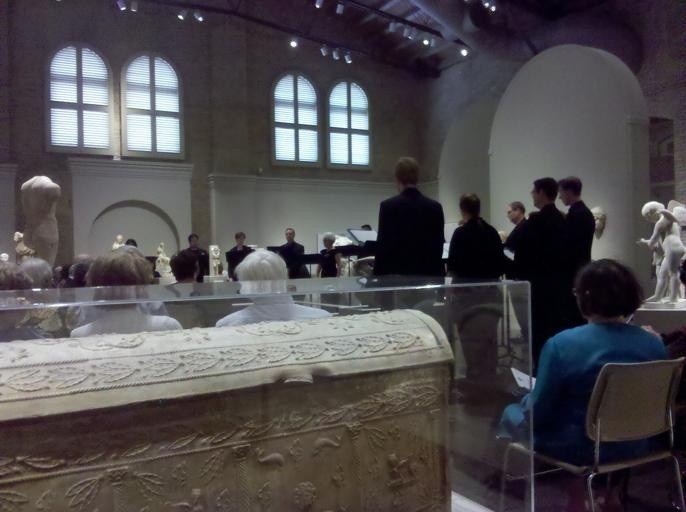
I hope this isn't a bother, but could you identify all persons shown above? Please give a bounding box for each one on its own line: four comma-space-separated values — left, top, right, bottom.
58, 264, 85, 302
446, 194, 503, 416
70, 252, 183, 338
20, 258, 67, 338
77, 246, 167, 326
503, 200, 529, 345
483, 261, 667, 499
590, 207, 606, 231
226, 232, 255, 282
113, 234, 126, 251
165, 251, 199, 296
516, 176, 565, 378
0, 263, 45, 342
357, 225, 376, 259
365, 157, 444, 313
316, 232, 342, 314
278, 227, 305, 280
51, 267, 63, 287
183, 234, 210, 283
635, 200, 686, 303
215, 250, 331, 328
558, 177, 596, 329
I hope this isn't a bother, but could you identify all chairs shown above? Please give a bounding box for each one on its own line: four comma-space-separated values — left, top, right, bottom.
497, 353, 686, 512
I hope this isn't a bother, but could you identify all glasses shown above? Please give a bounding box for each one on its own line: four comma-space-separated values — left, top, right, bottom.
572, 287, 580, 297
